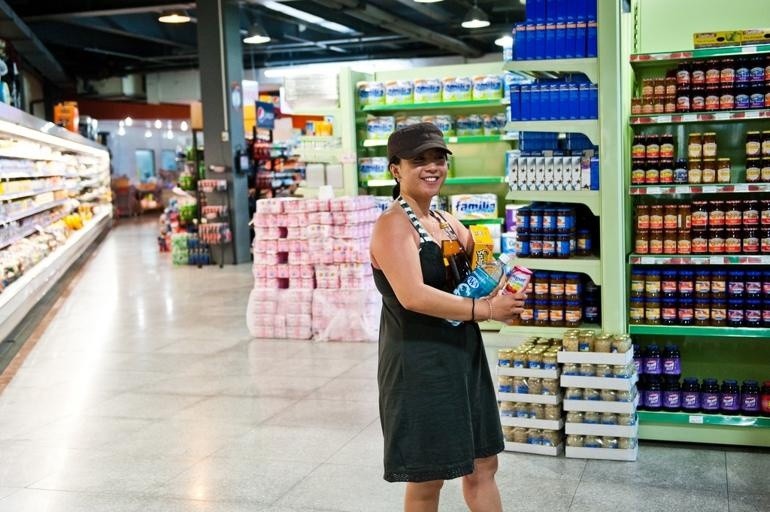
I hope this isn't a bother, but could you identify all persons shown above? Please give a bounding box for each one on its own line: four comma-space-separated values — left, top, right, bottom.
370, 123, 527, 512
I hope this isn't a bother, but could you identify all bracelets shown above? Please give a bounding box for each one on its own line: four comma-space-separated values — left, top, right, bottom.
485, 298, 493, 320
472, 297, 475, 321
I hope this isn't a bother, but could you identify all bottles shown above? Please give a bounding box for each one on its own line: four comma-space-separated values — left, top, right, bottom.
445, 253, 511, 326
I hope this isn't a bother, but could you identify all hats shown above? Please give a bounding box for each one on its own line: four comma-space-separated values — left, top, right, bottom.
387, 122, 452, 163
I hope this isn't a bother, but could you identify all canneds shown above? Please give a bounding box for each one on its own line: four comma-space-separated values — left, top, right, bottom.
629, 55, 770, 327
505, 202, 598, 328
497, 330, 770, 449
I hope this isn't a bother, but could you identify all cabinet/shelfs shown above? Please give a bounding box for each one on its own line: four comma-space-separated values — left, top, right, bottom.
0, 103, 115, 344
493, 0, 769, 465
278, 61, 508, 331
175, 83, 307, 270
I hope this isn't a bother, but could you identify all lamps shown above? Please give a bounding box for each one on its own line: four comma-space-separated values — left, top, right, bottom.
155, 11, 194, 28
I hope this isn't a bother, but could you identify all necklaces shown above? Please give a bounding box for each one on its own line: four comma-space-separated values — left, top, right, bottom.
430, 215, 435, 236
